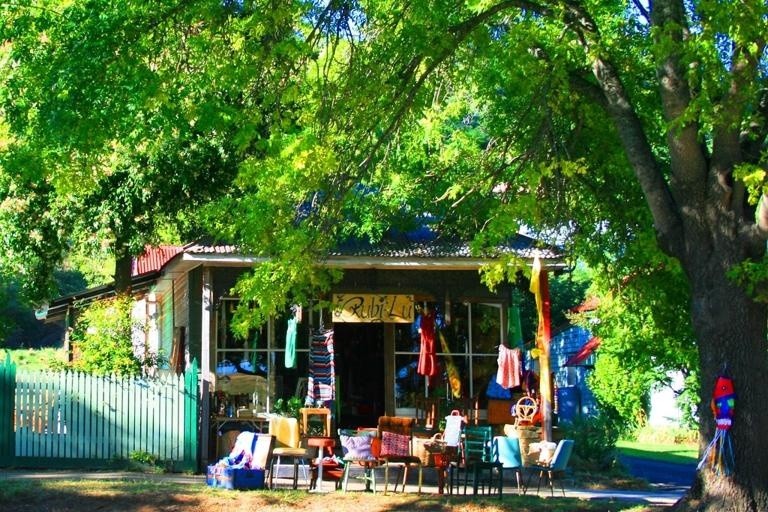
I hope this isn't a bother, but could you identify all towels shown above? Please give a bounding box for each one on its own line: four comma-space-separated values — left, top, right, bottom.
494, 340, 524, 393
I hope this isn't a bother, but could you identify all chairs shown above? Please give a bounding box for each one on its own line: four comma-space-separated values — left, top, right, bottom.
262, 393, 581, 502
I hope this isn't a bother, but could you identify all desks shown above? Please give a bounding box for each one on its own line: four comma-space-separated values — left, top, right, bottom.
208, 413, 270, 464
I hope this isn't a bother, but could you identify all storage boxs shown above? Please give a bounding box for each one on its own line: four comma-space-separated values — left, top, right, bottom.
203, 428, 278, 495
